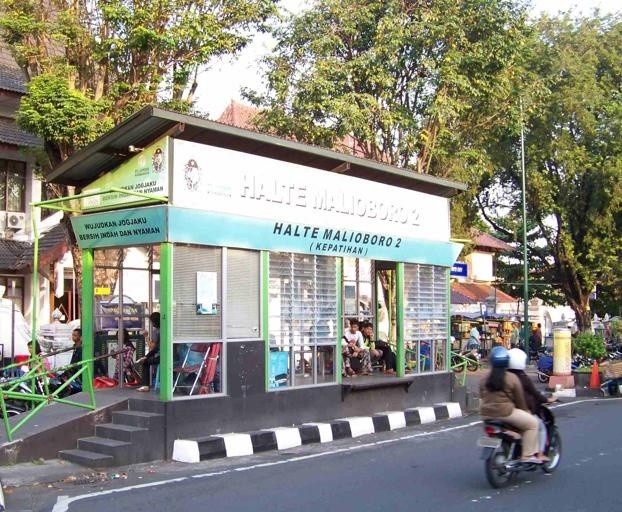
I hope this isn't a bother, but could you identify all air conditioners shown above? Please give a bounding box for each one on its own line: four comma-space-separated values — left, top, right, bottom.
6, 212, 24, 230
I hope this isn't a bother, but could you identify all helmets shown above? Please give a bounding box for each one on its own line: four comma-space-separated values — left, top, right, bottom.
489, 346, 509, 367
505, 348, 528, 370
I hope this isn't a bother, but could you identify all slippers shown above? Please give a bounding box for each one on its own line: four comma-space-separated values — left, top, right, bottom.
520, 455, 550, 464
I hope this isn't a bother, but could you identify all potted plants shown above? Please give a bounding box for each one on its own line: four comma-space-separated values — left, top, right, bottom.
572, 366, 604, 387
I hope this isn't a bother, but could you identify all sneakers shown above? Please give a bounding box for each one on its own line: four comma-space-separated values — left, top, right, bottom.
136, 386, 150, 391
342, 365, 410, 376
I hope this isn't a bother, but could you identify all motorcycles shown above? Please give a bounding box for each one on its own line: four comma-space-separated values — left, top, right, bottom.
451, 335, 622, 384
476, 384, 562, 489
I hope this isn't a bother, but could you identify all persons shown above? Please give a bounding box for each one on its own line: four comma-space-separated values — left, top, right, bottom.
68, 328, 82, 393
136, 312, 160, 392
528, 331, 541, 350
469, 323, 481, 352
479, 346, 543, 467
342, 320, 384, 377
25, 340, 55, 377
509, 321, 521, 348
508, 344, 559, 463
110, 328, 137, 383
535, 323, 543, 346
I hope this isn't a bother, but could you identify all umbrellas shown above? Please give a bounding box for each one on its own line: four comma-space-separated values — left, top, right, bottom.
197, 343, 221, 394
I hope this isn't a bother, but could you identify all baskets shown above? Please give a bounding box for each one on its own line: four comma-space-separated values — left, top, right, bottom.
599, 359, 622, 379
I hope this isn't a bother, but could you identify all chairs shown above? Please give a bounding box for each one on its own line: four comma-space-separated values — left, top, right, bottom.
149, 343, 210, 395
